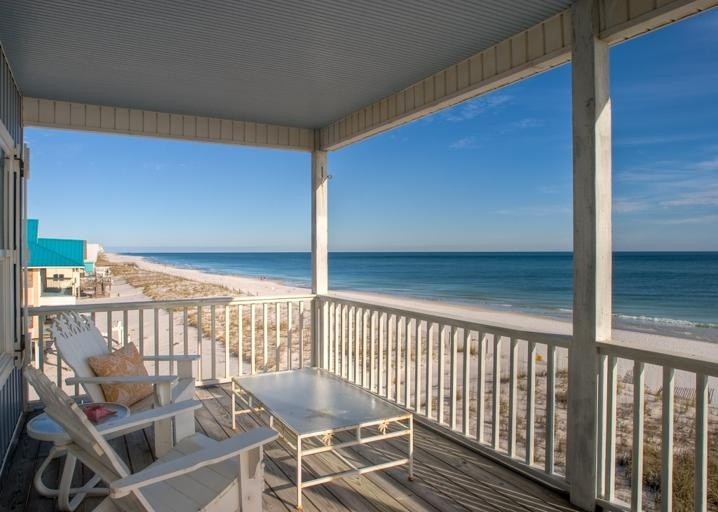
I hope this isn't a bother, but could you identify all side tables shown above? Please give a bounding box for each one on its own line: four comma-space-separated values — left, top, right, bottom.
19, 401, 132, 512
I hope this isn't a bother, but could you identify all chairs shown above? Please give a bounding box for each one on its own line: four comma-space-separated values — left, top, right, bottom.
18, 363, 280, 512
46, 308, 201, 446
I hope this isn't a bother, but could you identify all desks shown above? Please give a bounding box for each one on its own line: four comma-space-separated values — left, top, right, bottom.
231, 365, 416, 510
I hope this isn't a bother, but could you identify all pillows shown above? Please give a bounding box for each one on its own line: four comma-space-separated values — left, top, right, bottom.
86, 341, 154, 407
